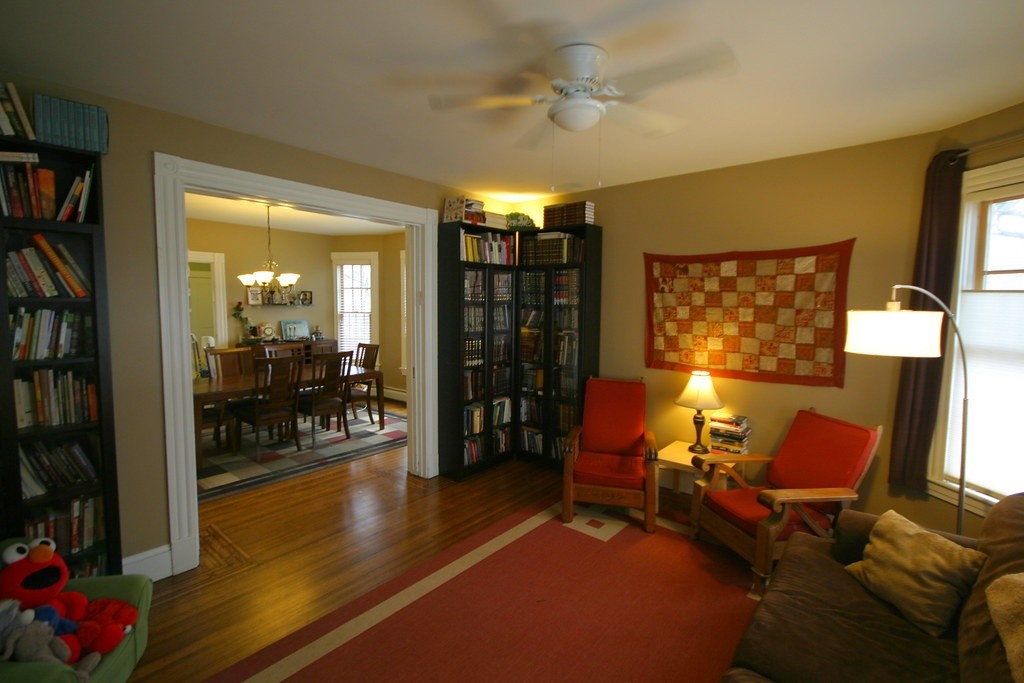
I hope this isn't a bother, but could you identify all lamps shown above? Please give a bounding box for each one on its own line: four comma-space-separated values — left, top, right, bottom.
674, 371, 724, 455
531, 44, 625, 132
236, 204, 301, 303
843, 284, 969, 534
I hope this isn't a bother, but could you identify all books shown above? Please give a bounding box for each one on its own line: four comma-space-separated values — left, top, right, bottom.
444, 195, 515, 467
709, 413, 752, 456
518, 232, 588, 462
0, 81, 109, 583
543, 200, 595, 227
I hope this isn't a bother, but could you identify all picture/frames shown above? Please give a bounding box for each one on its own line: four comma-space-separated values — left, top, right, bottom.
246, 287, 263, 306
300, 291, 313, 306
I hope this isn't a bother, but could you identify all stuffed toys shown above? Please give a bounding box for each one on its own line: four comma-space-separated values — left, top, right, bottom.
0, 540, 139, 683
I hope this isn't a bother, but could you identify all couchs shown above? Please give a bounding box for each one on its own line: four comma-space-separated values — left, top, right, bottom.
0, 567, 152, 683
720, 490, 1024, 683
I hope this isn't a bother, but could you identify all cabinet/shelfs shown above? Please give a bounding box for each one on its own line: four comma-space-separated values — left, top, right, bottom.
0, 134, 123, 585
437, 222, 602, 481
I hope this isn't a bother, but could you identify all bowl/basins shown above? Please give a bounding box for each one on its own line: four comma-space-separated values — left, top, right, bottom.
242, 337, 262, 345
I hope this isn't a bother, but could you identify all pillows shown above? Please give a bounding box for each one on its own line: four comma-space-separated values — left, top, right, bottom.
844, 509, 989, 638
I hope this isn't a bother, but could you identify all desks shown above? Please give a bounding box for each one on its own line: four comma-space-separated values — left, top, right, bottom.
193, 364, 385, 470
206, 339, 338, 376
648, 441, 745, 540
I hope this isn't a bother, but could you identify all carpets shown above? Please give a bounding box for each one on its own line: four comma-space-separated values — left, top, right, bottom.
195, 402, 407, 504
203, 491, 775, 683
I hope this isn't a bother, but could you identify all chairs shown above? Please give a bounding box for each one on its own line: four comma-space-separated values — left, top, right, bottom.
207, 343, 380, 456
561, 377, 662, 532
687, 409, 883, 596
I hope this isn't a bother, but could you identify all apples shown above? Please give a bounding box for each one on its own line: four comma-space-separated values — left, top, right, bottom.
247, 335, 255, 340
